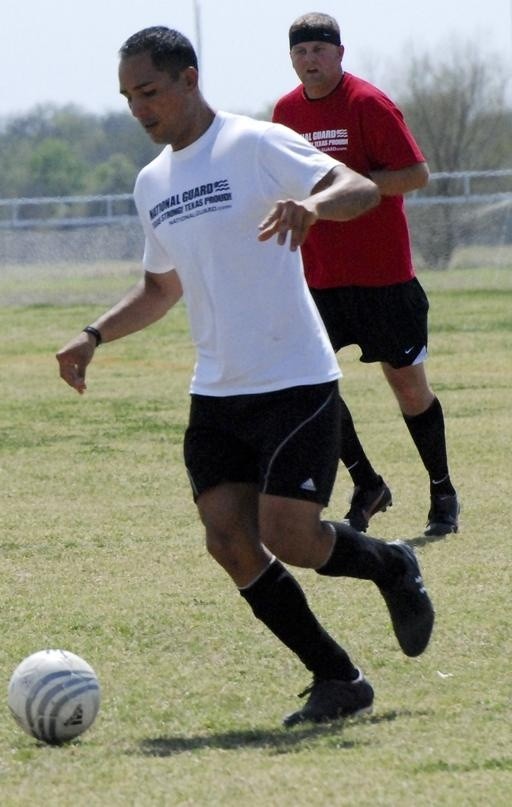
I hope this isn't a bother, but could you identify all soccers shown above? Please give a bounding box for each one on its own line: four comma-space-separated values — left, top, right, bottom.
8, 650, 98, 744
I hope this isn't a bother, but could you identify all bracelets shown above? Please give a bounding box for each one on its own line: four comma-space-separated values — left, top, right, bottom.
80, 324, 106, 349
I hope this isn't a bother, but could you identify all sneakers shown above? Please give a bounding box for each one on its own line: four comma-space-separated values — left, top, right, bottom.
284, 666, 374, 728
379, 539, 432, 656
344, 476, 391, 531
424, 493, 457, 538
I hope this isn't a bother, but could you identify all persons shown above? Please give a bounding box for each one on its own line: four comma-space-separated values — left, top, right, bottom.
54, 24, 435, 730
269, 11, 463, 540
